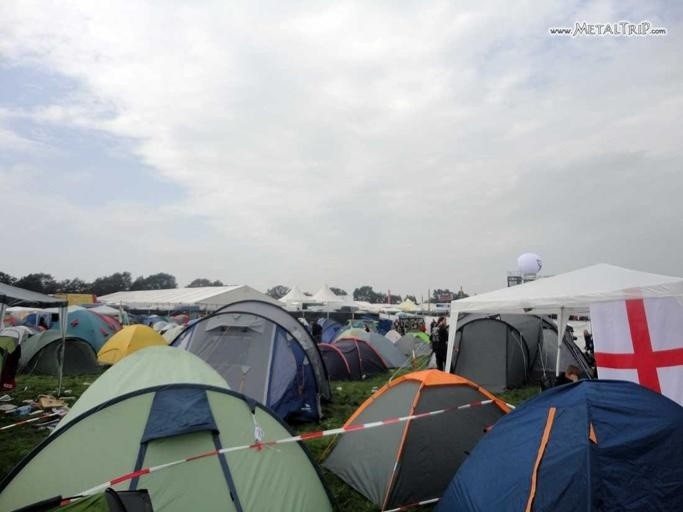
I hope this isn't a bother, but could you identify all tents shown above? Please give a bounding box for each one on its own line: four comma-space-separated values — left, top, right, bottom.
0, 298, 683, 512
